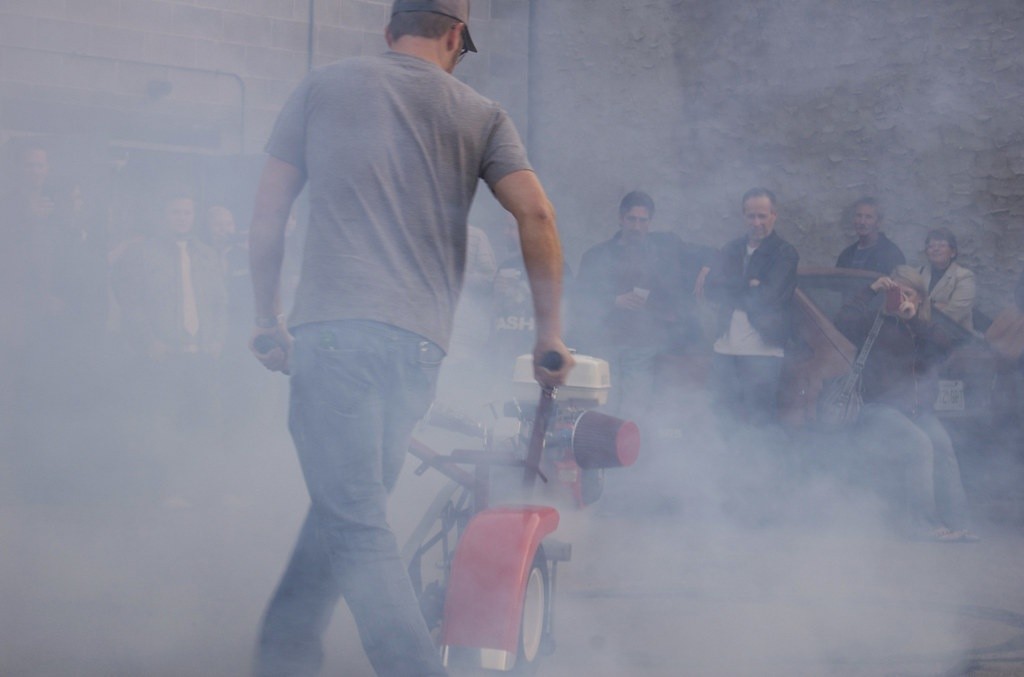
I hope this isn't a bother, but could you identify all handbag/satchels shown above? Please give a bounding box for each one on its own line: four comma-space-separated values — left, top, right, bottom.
819, 372, 864, 431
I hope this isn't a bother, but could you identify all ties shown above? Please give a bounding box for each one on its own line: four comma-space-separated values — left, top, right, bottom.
176, 239, 200, 336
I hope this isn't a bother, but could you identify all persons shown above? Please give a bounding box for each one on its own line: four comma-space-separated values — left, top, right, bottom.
244, 0, 575, 677
578, 188, 716, 420
913, 226, 977, 335
703, 186, 800, 432
487, 198, 604, 381
834, 264, 981, 544
835, 194, 907, 279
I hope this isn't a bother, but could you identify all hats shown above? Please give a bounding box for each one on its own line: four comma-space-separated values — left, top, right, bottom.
392, 0, 478, 54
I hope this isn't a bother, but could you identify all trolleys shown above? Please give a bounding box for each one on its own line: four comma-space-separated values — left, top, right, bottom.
252, 332, 640, 677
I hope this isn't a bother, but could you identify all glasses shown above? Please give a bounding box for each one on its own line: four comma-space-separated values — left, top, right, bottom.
927, 242, 950, 248
452, 19, 469, 65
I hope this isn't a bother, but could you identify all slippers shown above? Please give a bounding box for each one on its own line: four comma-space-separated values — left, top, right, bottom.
957, 534, 982, 541
913, 528, 963, 543
